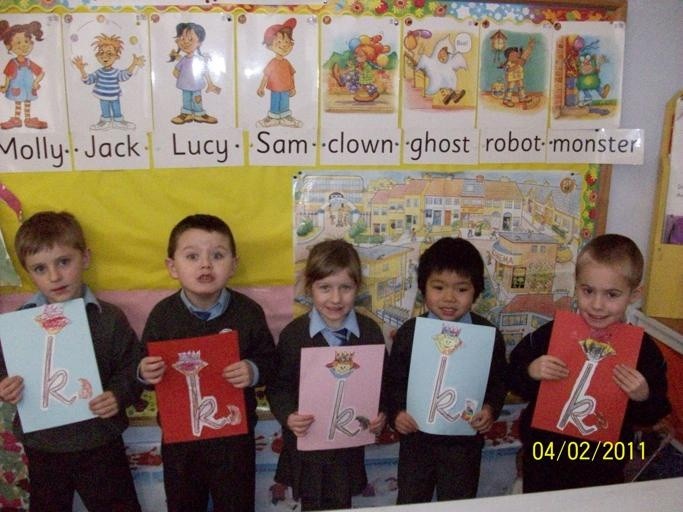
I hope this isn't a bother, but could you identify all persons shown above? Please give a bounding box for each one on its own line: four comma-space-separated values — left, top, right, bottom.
134, 214, 279, 512
386, 237, 509, 507
264, 239, 390, 511
508, 233, 671, 495
0, 210, 142, 510
